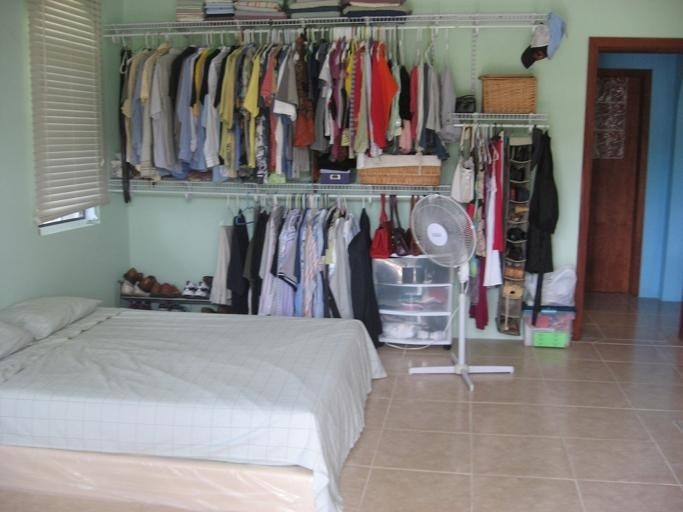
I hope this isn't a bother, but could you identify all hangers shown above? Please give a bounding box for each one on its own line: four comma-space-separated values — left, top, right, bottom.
223, 192, 365, 228
456, 121, 502, 168
111, 23, 449, 74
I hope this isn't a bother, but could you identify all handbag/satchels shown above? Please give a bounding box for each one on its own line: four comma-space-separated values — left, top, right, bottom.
455, 94, 476, 113
401, 267, 424, 284
387, 194, 410, 255
368, 194, 393, 259
449, 126, 476, 203
406, 194, 424, 256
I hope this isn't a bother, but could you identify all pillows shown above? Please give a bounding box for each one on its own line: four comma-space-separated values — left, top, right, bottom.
0, 294, 102, 343
0, 315, 36, 362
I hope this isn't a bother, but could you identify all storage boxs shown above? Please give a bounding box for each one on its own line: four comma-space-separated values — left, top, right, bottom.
520, 300, 577, 349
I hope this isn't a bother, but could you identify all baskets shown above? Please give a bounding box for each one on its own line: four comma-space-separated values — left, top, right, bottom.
355, 153, 444, 187
478, 73, 537, 115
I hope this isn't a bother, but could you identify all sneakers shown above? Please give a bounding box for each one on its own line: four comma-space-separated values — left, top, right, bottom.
120, 268, 210, 300
126, 299, 230, 313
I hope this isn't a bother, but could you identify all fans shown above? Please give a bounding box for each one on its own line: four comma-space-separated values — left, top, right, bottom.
406, 192, 515, 391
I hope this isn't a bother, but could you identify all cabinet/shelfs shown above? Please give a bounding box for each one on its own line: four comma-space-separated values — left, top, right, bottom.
97, 11, 551, 199
370, 252, 454, 352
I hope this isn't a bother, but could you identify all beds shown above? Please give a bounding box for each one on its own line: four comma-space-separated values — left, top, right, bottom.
0, 305, 390, 511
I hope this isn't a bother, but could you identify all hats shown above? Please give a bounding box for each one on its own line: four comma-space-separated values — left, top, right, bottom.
545, 12, 567, 59
520, 23, 551, 69
